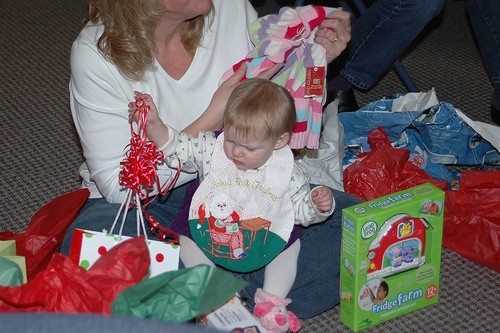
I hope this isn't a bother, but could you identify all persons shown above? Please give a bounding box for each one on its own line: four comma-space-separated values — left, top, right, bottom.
52, 0, 370, 329
257, 0, 500, 112
127, 79, 336, 333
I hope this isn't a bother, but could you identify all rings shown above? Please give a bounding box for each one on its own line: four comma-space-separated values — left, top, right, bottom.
330, 33, 338, 43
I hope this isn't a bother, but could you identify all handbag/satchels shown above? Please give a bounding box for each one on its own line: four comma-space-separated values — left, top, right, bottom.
69, 100, 181, 282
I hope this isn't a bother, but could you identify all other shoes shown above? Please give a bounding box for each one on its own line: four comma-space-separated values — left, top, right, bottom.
248, 287, 301, 333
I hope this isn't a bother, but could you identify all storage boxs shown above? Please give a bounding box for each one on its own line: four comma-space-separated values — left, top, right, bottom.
340, 182, 445, 331
